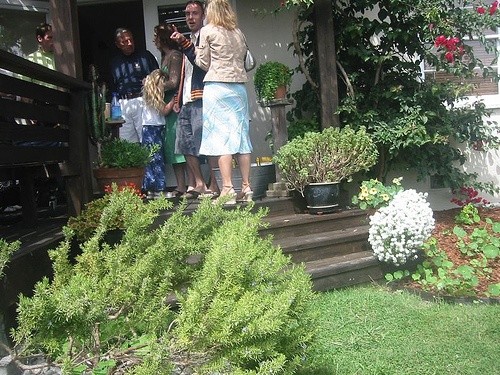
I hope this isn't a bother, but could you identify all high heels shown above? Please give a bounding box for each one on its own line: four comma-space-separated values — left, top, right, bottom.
211, 183, 237, 205
238, 182, 254, 202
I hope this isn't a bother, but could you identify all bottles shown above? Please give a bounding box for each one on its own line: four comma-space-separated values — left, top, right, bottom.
110, 92, 121, 120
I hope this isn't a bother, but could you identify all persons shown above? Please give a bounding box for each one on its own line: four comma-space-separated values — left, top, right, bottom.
166, 0, 220, 200
186, 0, 254, 204
23, 24, 60, 197
149, 25, 196, 200
139, 69, 176, 200
103, 28, 158, 145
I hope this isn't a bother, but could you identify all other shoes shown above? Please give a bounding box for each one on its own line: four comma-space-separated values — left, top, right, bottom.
147, 191, 173, 206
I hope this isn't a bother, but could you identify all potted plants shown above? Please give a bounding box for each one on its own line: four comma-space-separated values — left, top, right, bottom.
86, 137, 161, 197
253, 60, 295, 102
272, 124, 380, 214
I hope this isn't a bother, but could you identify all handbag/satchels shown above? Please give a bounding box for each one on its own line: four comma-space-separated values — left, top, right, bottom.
243, 49, 256, 72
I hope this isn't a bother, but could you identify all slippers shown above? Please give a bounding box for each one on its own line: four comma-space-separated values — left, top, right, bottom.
197, 189, 221, 200
181, 190, 201, 199
172, 190, 183, 198
